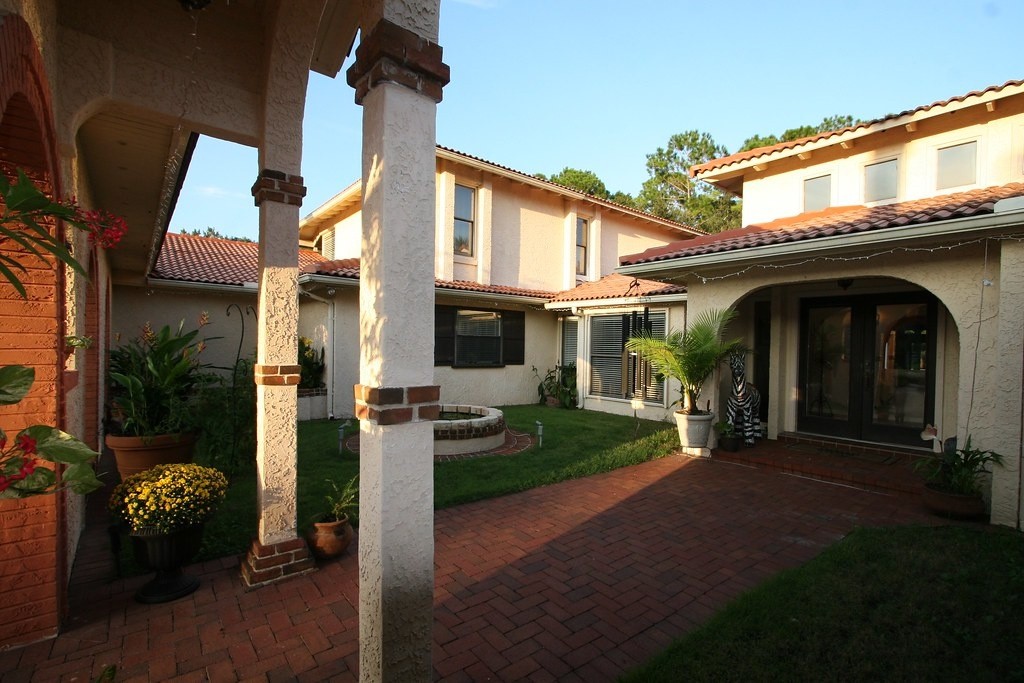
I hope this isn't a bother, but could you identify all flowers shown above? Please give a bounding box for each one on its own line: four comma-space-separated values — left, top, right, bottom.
297, 334, 326, 388
105, 307, 234, 436
104, 461, 231, 531
909, 423, 1009, 494
1, 158, 126, 506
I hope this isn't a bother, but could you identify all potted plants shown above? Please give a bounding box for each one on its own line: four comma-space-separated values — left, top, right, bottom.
623, 305, 756, 446
305, 472, 360, 559
528, 359, 578, 409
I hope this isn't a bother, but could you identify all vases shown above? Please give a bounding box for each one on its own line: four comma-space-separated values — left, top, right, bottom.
916, 478, 985, 515
123, 523, 209, 603
102, 430, 205, 484
